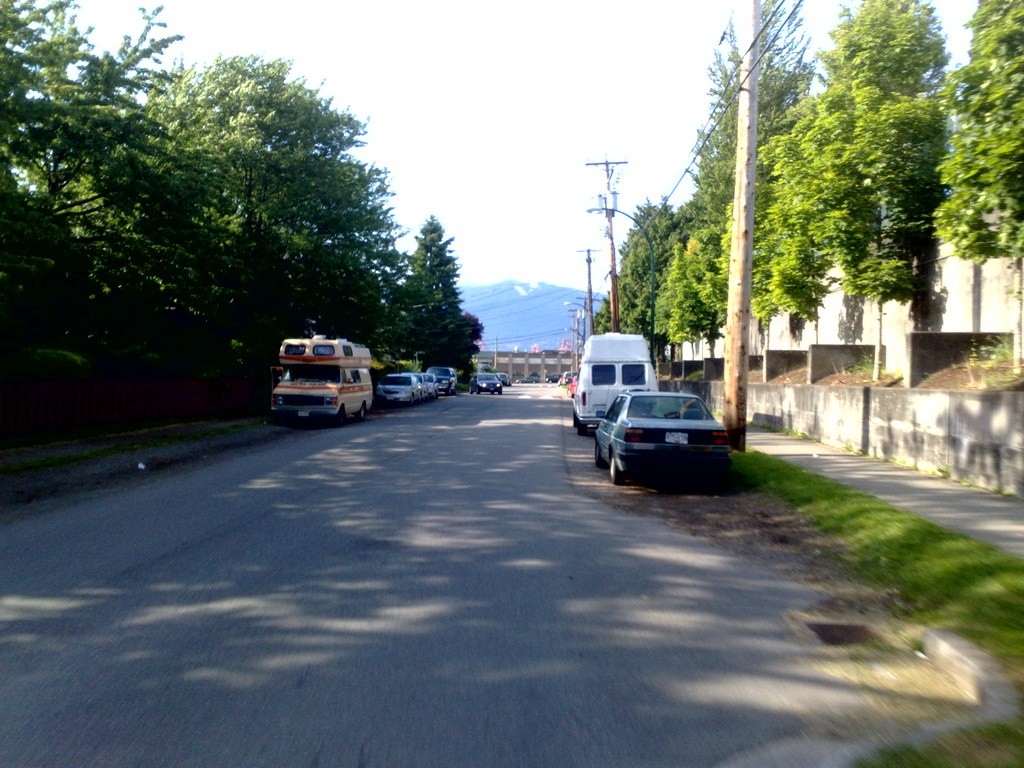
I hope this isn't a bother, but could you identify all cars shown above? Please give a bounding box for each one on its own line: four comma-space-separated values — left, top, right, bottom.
494, 372, 510, 386
468, 373, 503, 395
545, 371, 578, 398
515, 376, 540, 384
376, 372, 439, 407
592, 387, 732, 493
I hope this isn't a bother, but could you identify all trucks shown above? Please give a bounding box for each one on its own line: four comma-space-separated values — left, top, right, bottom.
269, 335, 375, 426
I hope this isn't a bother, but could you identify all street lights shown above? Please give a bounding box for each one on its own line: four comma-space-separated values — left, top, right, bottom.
587, 207, 655, 366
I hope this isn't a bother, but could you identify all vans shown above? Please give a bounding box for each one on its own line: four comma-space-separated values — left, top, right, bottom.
569, 332, 660, 436
425, 366, 458, 396
